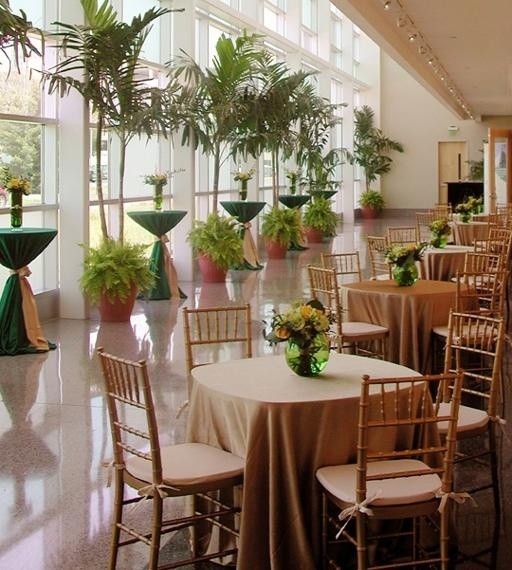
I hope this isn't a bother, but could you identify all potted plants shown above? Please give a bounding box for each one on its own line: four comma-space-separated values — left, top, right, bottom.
264, 206, 303, 259
73, 237, 161, 324
188, 216, 245, 281
359, 188, 386, 219
302, 198, 335, 246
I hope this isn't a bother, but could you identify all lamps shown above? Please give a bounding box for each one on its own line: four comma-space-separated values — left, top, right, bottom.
408, 30, 421, 42
397, 12, 409, 28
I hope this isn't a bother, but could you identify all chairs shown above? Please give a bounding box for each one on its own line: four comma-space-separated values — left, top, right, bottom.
488, 202, 512, 269
306, 263, 387, 362
367, 235, 395, 280
314, 366, 463, 570
425, 271, 505, 396
472, 238, 489, 253
321, 251, 362, 326
415, 200, 452, 235
387, 225, 419, 250
429, 307, 505, 513
451, 253, 502, 318
181, 303, 252, 408
97, 346, 245, 570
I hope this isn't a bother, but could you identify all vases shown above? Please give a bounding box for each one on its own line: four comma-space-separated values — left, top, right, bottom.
430, 230, 447, 248
241, 182, 248, 201
10, 193, 24, 226
393, 262, 419, 287
153, 185, 163, 209
474, 205, 482, 214
458, 211, 472, 224
285, 332, 330, 377
290, 179, 297, 194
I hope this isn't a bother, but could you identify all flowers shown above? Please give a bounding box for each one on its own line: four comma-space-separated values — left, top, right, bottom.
469, 193, 482, 212
1, 161, 31, 223
262, 299, 338, 374
285, 169, 302, 194
233, 167, 256, 199
454, 203, 472, 220
428, 219, 452, 247
141, 169, 186, 209
381, 242, 426, 283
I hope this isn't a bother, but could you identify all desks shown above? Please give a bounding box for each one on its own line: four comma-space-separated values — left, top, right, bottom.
184, 353, 445, 570
445, 219, 495, 245
217, 201, 269, 270
413, 245, 485, 281
279, 194, 311, 250
310, 189, 337, 239
0, 226, 58, 355
125, 210, 188, 299
450, 212, 499, 223
339, 280, 481, 392
